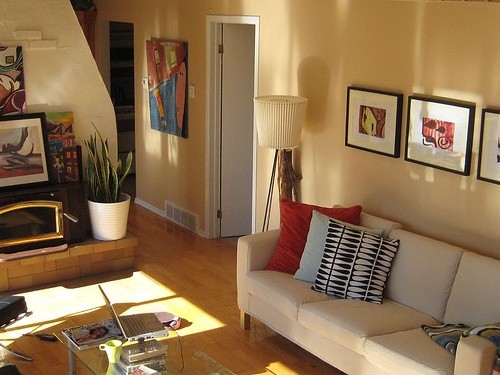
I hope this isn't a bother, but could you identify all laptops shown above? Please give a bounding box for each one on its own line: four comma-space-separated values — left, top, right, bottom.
98, 285, 168, 340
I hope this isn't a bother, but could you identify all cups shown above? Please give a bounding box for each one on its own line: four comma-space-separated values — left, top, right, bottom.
99, 340, 122, 363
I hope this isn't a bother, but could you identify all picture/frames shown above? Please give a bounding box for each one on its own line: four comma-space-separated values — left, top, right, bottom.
476, 108, 500, 185
404, 96, 475, 176
0, 112, 54, 193
345, 86, 402, 158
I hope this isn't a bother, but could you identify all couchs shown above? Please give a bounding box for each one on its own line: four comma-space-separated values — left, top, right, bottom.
237, 204, 500, 375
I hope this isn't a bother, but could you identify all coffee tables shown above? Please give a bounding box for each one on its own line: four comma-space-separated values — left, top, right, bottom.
52, 319, 235, 375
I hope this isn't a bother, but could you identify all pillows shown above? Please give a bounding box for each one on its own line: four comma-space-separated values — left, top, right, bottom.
293, 210, 384, 283
311, 218, 400, 305
265, 200, 363, 275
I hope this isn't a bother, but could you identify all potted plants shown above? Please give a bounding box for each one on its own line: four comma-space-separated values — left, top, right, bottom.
77, 121, 132, 240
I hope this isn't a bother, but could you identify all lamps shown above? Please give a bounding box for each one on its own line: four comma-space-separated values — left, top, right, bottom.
252, 95, 308, 232
